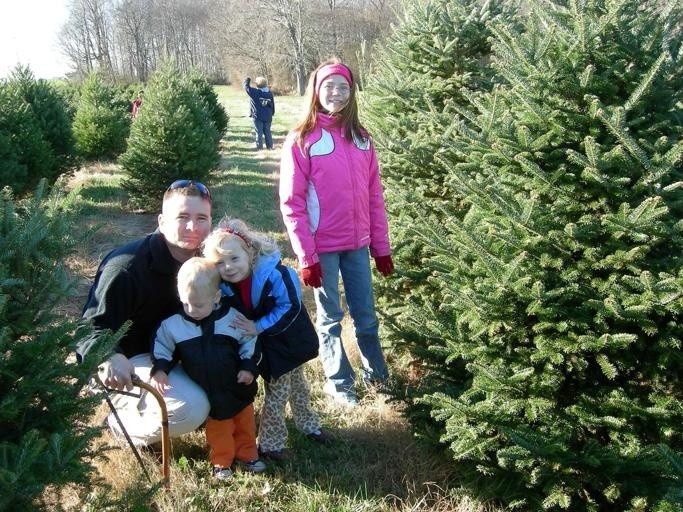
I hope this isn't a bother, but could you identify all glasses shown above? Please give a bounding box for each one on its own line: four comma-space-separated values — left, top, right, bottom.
166, 180, 210, 199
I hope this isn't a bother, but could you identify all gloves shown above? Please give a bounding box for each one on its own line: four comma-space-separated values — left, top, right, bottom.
301, 263, 323, 289
373, 255, 394, 277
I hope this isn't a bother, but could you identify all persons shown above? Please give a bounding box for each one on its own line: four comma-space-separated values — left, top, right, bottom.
132, 89, 144, 117
74, 178, 213, 453
243, 76, 275, 150
199, 213, 338, 461
147, 256, 266, 484
278, 56, 393, 406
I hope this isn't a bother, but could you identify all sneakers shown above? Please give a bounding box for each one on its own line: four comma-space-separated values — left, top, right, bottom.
211, 465, 232, 482
309, 428, 335, 446
324, 380, 357, 406
265, 449, 293, 463
234, 457, 265, 474
